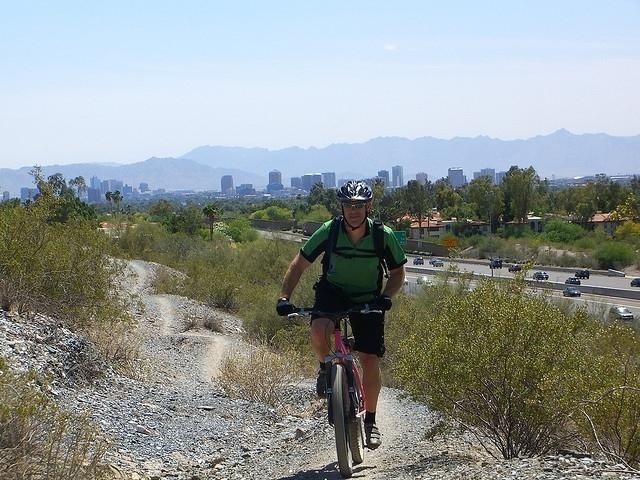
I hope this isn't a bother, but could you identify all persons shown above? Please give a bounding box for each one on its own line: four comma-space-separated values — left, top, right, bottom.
278, 178, 406, 450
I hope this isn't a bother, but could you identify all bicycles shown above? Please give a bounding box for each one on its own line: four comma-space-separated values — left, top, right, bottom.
282, 303, 389, 477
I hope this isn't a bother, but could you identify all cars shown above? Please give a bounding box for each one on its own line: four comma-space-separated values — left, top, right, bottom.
413, 256, 444, 268
489, 259, 590, 298
631, 277, 640, 288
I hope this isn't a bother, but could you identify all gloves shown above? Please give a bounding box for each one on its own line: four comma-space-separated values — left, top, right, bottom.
276, 297, 296, 316
376, 295, 392, 311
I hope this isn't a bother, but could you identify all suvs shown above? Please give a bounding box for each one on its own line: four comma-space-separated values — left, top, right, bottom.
609, 306, 634, 322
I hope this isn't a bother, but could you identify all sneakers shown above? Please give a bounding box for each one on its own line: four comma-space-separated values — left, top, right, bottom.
316, 361, 332, 398
364, 423, 381, 448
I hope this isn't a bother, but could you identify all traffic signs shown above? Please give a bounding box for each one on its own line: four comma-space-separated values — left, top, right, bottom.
393, 231, 407, 245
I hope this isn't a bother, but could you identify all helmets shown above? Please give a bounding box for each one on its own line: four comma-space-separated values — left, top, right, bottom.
336, 180, 373, 202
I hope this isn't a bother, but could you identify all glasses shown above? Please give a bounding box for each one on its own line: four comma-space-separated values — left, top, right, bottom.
342, 201, 366, 209
